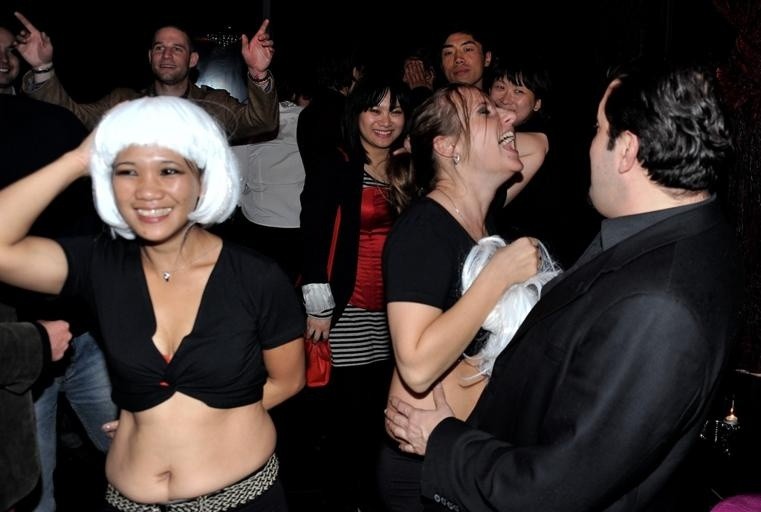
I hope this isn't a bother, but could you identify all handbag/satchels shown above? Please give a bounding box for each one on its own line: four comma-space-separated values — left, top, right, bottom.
302, 327, 334, 388
